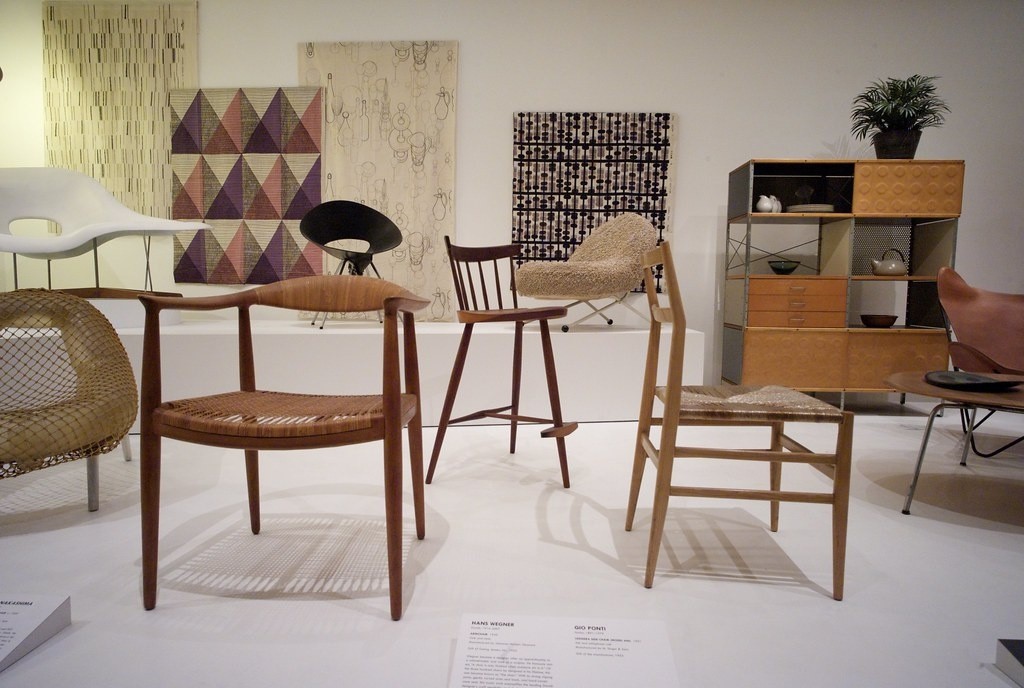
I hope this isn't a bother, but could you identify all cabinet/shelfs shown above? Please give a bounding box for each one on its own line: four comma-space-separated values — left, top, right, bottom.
720, 158, 966, 418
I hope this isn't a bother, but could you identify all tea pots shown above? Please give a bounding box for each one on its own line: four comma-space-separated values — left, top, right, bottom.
870, 248, 907, 276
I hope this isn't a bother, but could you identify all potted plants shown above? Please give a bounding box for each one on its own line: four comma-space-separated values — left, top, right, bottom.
850, 72, 952, 160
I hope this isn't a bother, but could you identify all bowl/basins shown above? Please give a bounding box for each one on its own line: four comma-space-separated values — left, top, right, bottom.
767, 261, 799, 275
859, 314, 898, 327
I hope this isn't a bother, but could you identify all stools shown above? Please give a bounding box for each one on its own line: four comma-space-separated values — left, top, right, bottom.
0, 167, 215, 462
300, 201, 403, 330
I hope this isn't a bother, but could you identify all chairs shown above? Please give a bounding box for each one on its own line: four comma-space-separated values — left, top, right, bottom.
425, 236, 579, 488
0, 287, 139, 513
625, 239, 855, 602
901, 267, 1024, 516
515, 212, 663, 333
136, 275, 433, 622
